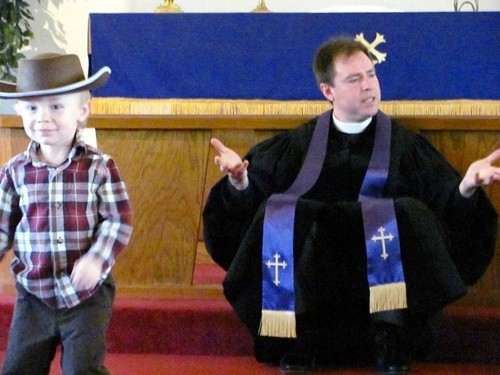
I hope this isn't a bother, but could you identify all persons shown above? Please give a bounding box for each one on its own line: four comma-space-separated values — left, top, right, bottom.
200, 37, 500, 375
0, 52, 132, 375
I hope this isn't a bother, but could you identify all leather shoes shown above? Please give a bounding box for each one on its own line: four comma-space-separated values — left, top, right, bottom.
375, 346, 412, 374
280, 350, 315, 375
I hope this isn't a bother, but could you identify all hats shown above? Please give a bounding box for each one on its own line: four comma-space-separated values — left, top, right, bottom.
0, 51, 113, 99
